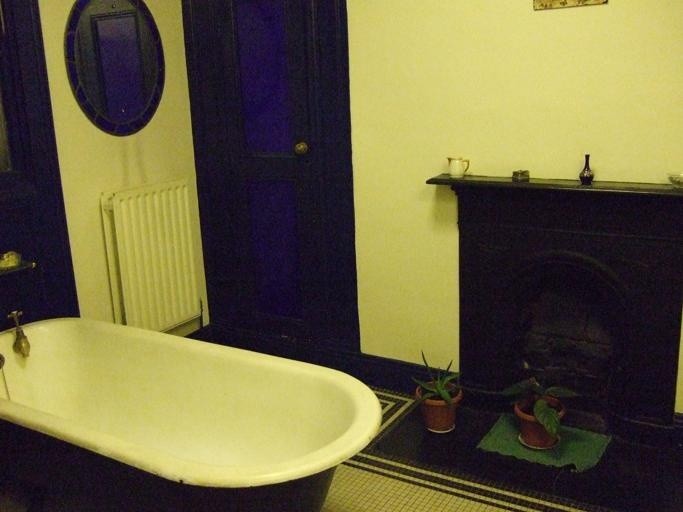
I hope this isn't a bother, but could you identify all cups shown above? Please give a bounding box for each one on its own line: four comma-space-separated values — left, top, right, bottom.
447, 156, 470, 180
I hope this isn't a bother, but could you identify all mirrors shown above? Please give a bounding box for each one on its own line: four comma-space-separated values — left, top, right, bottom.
66, 0, 165, 139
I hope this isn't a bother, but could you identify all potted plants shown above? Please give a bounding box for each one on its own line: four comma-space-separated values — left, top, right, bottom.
499, 378, 585, 450
410, 350, 464, 434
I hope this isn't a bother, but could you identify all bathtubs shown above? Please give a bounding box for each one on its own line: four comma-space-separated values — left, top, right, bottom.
1, 316, 382, 490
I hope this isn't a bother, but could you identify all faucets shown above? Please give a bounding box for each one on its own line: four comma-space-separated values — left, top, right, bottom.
5, 310, 34, 359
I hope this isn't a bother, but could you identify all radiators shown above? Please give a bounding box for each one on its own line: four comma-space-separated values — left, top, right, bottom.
99, 175, 202, 333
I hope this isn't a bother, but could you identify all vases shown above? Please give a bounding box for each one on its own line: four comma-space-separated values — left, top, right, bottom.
578, 154, 594, 185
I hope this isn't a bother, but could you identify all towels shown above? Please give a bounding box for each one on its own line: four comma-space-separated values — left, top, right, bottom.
475, 413, 614, 474
